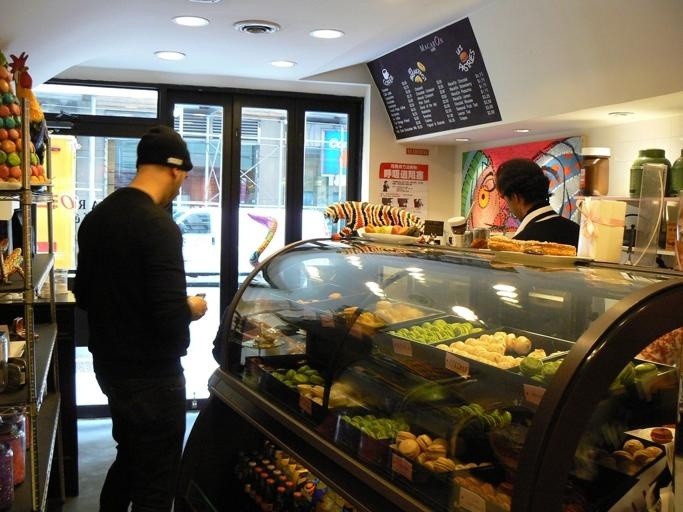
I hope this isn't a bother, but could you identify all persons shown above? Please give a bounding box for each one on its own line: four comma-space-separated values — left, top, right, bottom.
494, 156, 582, 249
382, 180, 389, 191
71, 125, 208, 512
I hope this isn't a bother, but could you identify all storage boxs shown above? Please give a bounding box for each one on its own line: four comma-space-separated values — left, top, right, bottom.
577, 199, 627, 263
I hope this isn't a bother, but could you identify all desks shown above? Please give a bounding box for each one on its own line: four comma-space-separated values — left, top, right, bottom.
1, 273, 79, 499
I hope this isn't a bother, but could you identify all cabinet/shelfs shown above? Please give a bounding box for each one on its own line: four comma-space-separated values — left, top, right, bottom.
575, 193, 681, 257
1, 98, 67, 511
173, 234, 683, 512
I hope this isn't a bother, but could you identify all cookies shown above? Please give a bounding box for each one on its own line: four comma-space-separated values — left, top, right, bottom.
0, 52, 47, 191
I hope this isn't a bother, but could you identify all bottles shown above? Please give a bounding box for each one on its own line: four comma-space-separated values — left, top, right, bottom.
578, 145, 611, 197
232, 437, 356, 511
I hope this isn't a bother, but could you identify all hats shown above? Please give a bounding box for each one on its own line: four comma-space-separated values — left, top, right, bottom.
136, 125, 193, 171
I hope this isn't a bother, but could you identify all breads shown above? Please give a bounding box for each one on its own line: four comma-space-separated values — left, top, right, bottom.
267, 364, 384, 409
335, 404, 514, 511
320, 298, 547, 370
514, 357, 562, 385
484, 234, 578, 257
572, 361, 673, 484
